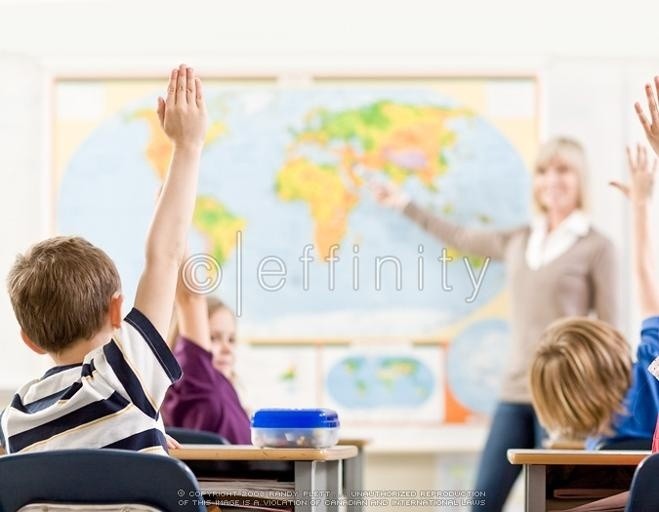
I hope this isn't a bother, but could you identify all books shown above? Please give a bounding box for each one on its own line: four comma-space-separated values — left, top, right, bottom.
195, 473, 294, 512
546, 481, 625, 503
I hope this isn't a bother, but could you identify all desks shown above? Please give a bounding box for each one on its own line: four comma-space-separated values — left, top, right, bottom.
505, 448, 652, 512
136, 440, 359, 512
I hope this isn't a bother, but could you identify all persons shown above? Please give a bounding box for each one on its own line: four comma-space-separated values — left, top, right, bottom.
369, 129, 625, 512
160, 234, 256, 447
2, 63, 210, 460
631, 76, 658, 459
524, 139, 658, 448
